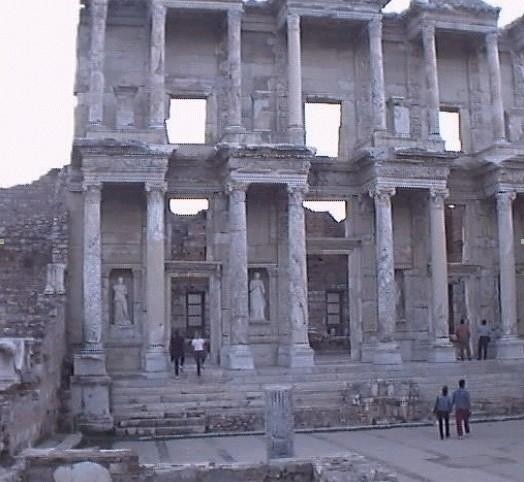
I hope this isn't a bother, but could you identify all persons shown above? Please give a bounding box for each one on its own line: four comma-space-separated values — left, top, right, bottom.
190, 330, 207, 376
474, 319, 492, 359
111, 276, 132, 327
455, 318, 472, 362
169, 328, 184, 377
450, 379, 472, 440
248, 271, 267, 320
432, 385, 452, 440
